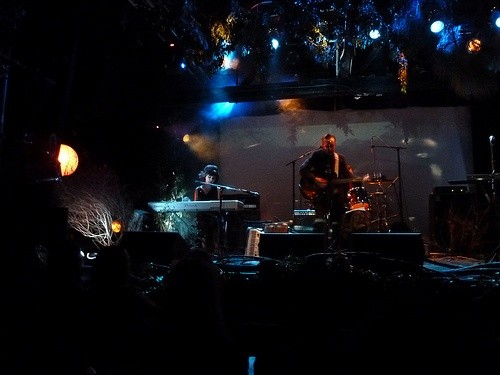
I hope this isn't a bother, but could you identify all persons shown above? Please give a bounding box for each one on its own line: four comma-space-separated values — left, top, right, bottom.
194, 164, 228, 257
300, 133, 371, 260
352, 181, 368, 201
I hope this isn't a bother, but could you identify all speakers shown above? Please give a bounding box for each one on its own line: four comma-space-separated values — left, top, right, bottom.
350, 233, 426, 260
259, 233, 328, 258
112, 231, 189, 256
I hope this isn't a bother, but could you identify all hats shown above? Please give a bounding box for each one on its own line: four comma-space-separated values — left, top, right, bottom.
322, 134, 336, 146
203, 165, 219, 176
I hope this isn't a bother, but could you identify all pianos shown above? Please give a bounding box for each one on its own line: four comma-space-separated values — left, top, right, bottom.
147, 200, 244, 212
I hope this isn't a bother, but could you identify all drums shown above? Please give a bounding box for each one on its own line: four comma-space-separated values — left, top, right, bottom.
294, 209, 316, 227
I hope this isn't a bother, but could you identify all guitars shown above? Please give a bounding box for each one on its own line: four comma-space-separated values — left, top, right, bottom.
299, 170, 384, 200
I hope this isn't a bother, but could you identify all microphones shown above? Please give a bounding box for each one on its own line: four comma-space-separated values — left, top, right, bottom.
489, 136, 495, 144
371, 137, 373, 153
195, 180, 210, 186
315, 146, 323, 150
